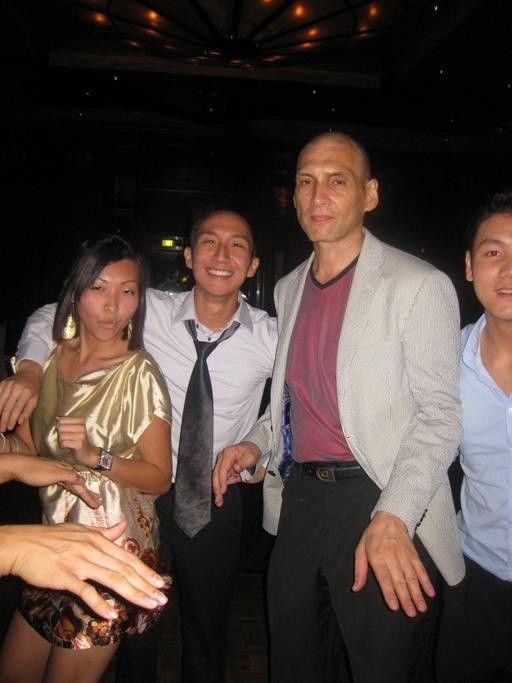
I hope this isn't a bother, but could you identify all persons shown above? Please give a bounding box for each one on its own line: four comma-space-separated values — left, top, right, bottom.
435, 190, 512, 682
2, 207, 277, 683
2, 236, 174, 683
2, 452, 171, 620
211, 132, 465, 682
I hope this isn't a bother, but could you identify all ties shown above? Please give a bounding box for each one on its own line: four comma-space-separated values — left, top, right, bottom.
174, 320, 241, 539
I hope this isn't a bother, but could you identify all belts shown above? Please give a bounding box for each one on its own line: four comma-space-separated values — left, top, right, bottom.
293, 463, 364, 480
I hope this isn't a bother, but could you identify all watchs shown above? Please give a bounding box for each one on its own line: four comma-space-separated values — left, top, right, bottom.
91, 450, 113, 473
